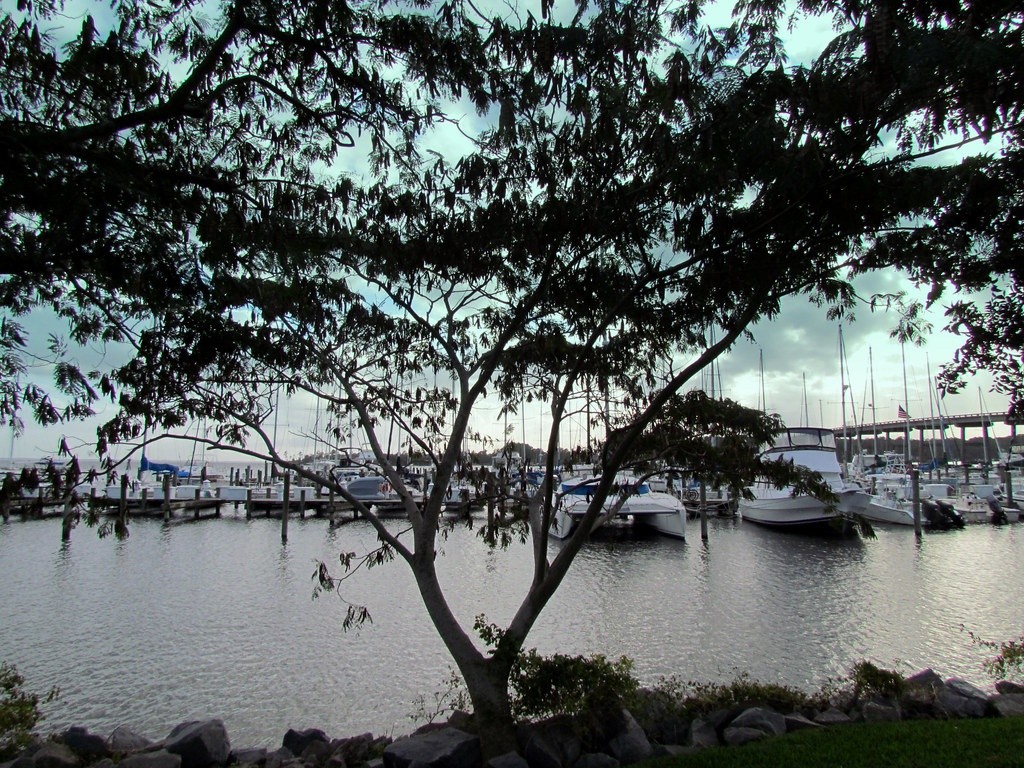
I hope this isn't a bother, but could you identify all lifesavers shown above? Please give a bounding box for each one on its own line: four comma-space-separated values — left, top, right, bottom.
381, 482, 390, 494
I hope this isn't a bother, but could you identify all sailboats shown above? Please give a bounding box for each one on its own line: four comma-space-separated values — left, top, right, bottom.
0, 309, 1024, 541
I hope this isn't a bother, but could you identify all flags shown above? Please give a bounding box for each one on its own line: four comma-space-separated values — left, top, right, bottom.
897, 405, 912, 418
